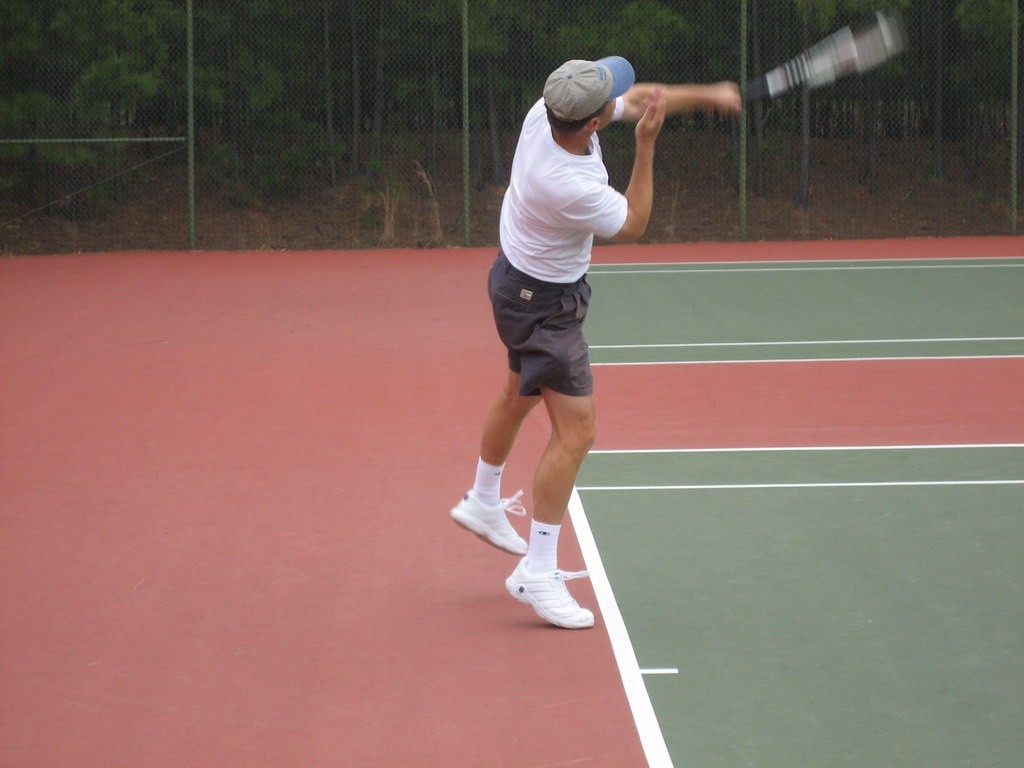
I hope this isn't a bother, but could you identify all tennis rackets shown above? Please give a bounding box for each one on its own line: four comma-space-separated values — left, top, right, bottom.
740, 10, 908, 105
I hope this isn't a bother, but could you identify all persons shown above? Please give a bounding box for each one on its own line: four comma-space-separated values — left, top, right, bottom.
447, 56, 744, 628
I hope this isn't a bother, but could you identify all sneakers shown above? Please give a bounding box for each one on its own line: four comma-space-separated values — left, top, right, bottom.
450, 489, 530, 556
505, 557, 595, 629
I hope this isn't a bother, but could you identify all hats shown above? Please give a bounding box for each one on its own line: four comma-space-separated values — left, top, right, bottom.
542, 55, 636, 122
